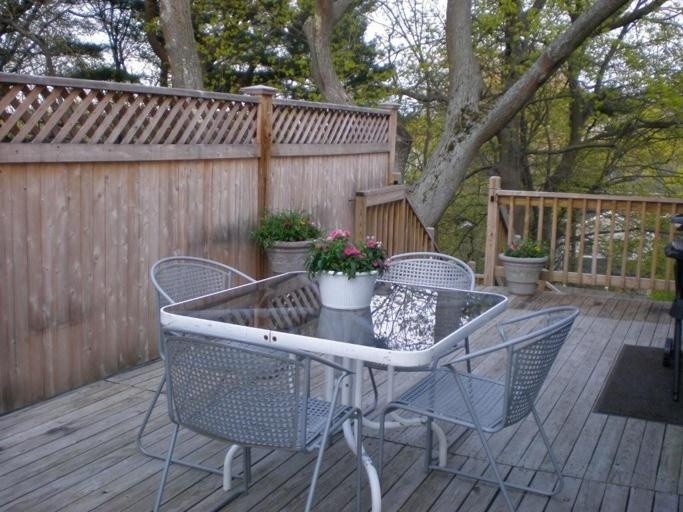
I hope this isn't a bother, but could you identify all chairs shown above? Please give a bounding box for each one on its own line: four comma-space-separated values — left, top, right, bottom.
133, 254, 268, 482
375, 304, 583, 512
344, 248, 481, 462
151, 323, 367, 512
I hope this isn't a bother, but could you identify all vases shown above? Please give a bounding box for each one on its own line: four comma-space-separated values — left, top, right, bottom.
496, 252, 549, 297
313, 268, 377, 311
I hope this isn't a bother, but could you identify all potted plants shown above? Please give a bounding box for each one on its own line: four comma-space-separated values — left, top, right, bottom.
246, 208, 323, 275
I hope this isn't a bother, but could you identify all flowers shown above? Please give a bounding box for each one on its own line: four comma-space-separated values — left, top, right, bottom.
297, 227, 388, 282
501, 234, 550, 257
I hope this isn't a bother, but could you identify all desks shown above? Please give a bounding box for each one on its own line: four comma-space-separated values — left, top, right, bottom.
155, 269, 512, 512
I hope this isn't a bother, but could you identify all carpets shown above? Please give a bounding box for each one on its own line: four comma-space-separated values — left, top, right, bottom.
591, 342, 683, 427
